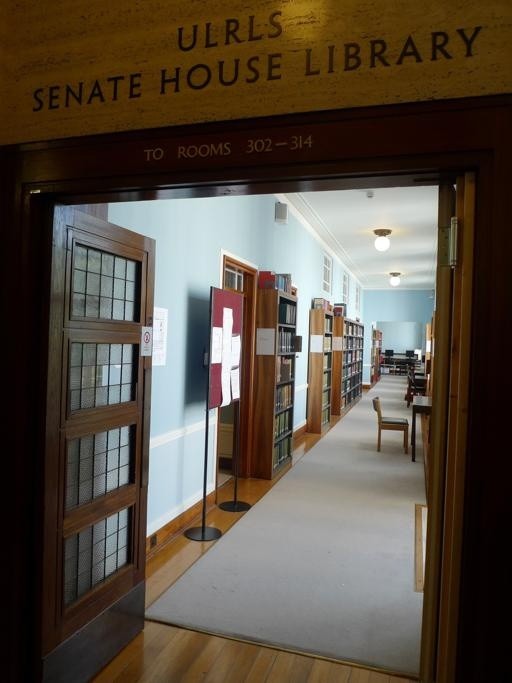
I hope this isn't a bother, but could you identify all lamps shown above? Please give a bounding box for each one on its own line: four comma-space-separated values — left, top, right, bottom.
373, 229, 392, 251
389, 272, 400, 286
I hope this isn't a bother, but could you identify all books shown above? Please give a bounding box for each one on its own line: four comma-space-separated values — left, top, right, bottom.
271, 303, 297, 470
258, 270, 292, 295
313, 298, 364, 425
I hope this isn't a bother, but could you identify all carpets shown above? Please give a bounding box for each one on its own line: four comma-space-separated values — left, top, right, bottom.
144, 375, 426, 679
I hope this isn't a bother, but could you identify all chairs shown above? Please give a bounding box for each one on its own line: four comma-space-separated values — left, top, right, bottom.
406, 364, 426, 408
373, 396, 408, 454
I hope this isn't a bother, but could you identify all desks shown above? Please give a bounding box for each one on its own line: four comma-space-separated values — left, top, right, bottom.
411, 395, 432, 462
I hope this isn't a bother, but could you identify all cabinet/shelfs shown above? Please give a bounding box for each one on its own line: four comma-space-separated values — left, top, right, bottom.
372, 330, 383, 383
306, 308, 364, 434
240, 287, 297, 480
380, 363, 406, 376
